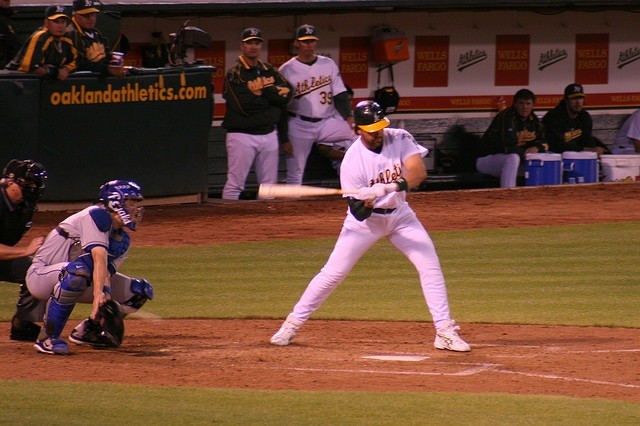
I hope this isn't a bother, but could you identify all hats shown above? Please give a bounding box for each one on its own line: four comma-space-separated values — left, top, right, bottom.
241, 28, 263, 41
45, 5, 69, 20
72, 0, 100, 15
297, 24, 318, 41
565, 84, 586, 98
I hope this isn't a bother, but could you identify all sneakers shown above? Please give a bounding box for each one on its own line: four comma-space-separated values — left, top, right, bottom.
434, 319, 470, 352
32, 334, 68, 354
10, 316, 41, 340
270, 315, 304, 346
69, 328, 112, 347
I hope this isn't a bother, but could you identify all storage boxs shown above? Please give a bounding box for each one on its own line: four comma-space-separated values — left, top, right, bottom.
599, 155, 640, 182
563, 151, 598, 184
524, 153, 563, 186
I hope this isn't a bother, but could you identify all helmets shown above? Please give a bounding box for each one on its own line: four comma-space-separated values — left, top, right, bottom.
2, 158, 47, 211
354, 100, 390, 133
99, 179, 144, 230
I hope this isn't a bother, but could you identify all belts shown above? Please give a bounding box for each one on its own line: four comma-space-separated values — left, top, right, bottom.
289, 112, 323, 122
371, 208, 397, 214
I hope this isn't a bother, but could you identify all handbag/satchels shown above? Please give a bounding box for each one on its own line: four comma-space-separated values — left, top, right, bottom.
375, 87, 399, 114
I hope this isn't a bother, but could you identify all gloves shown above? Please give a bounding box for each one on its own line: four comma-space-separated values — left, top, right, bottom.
371, 183, 397, 196
356, 187, 376, 199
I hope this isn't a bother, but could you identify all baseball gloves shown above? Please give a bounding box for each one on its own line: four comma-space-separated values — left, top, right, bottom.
96, 300, 126, 348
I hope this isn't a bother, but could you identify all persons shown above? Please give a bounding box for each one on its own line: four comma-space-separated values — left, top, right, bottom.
66, 0, 129, 77
221, 27, 293, 199
0, 0, 20, 69
0, 159, 45, 341
316, 84, 358, 175
267, 98, 471, 353
541, 83, 612, 155
23, 180, 154, 356
611, 108, 640, 155
4, 5, 77, 79
277, 24, 358, 186
474, 89, 549, 187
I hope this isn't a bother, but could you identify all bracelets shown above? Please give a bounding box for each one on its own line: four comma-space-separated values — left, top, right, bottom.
393, 176, 408, 192
102, 285, 112, 295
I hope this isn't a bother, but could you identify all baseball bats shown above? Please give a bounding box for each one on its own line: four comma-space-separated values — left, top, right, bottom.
258, 183, 359, 201
